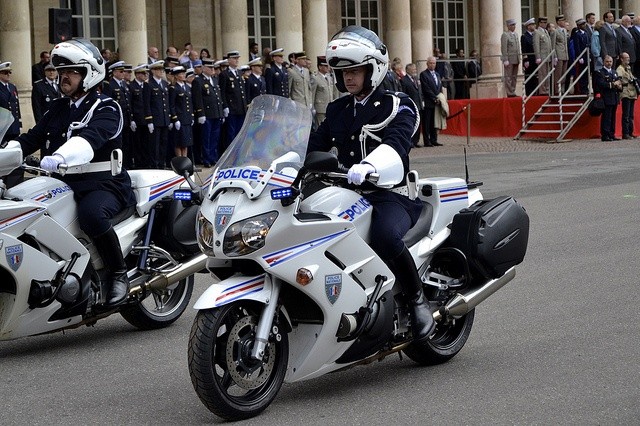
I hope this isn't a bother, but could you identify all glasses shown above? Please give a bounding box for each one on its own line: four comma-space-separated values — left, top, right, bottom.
58, 68, 80, 75
1, 71, 12, 74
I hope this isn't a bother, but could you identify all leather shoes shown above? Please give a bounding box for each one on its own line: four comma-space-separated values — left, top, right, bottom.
212, 161, 217, 165
426, 144, 434, 147
611, 138, 620, 140
630, 134, 637, 138
415, 144, 422, 148
622, 134, 633, 139
411, 142, 414, 148
434, 142, 443, 146
204, 164, 211, 168
602, 138, 613, 141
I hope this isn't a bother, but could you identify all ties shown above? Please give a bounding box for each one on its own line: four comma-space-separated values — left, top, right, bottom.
70, 104, 76, 116
354, 103, 364, 117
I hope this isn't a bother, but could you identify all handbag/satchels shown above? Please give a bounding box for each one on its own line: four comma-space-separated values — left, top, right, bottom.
589, 98, 605, 116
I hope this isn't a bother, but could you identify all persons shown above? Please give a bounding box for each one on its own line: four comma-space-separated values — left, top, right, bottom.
599, 12, 617, 68
552, 14, 569, 93
0, 40, 138, 309
392, 58, 405, 80
31, 64, 62, 150
420, 56, 443, 147
521, 18, 537, 95
629, 16, 639, 90
500, 19, 520, 97
433, 47, 441, 59
103, 42, 342, 163
614, 15, 636, 81
583, 13, 596, 94
545, 22, 555, 35
594, 56, 620, 141
467, 49, 483, 99
399, 63, 425, 147
569, 27, 581, 95
1, 61, 24, 145
532, 16, 553, 95
564, 21, 571, 38
268, 24, 436, 341
436, 53, 454, 100
574, 19, 591, 98
591, 20, 604, 94
450, 49, 470, 99
378, 58, 402, 92
30, 50, 51, 83
615, 53, 640, 140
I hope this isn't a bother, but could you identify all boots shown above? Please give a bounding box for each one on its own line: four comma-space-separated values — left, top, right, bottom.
90, 222, 129, 308
386, 242, 436, 342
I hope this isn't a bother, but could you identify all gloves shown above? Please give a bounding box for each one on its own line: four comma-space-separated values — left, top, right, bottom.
503, 60, 509, 66
222, 118, 225, 123
129, 121, 137, 132
579, 58, 584, 64
310, 104, 312, 109
40, 153, 65, 173
524, 61, 529, 68
347, 163, 376, 185
312, 109, 317, 116
191, 120, 195, 126
168, 123, 173, 130
197, 115, 206, 124
223, 107, 229, 117
174, 120, 181, 131
147, 123, 155, 133
554, 58, 558, 66
535, 58, 541, 64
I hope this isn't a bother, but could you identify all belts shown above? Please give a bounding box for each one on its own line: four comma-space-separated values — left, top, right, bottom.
65, 161, 112, 175
355, 185, 409, 198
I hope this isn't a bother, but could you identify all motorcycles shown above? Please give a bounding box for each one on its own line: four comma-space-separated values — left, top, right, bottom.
170, 93, 531, 422
0, 106, 209, 342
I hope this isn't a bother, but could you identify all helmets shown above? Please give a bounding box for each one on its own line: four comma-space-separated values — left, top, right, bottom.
51, 38, 107, 94
326, 25, 389, 92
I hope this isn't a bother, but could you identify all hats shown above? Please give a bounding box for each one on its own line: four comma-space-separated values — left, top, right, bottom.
166, 56, 179, 64
192, 60, 202, 67
186, 67, 195, 78
0, 62, 12, 71
506, 18, 516, 25
248, 56, 263, 66
124, 64, 133, 71
217, 59, 229, 65
227, 50, 240, 58
556, 14, 564, 18
524, 17, 535, 26
171, 65, 187, 74
202, 59, 215, 66
240, 64, 251, 71
317, 56, 328, 65
576, 18, 586, 24
165, 66, 172, 73
294, 51, 307, 59
45, 64, 55, 70
149, 60, 165, 69
538, 17, 547, 20
133, 64, 150, 72
269, 48, 285, 55
627, 12, 634, 17
108, 61, 125, 71
